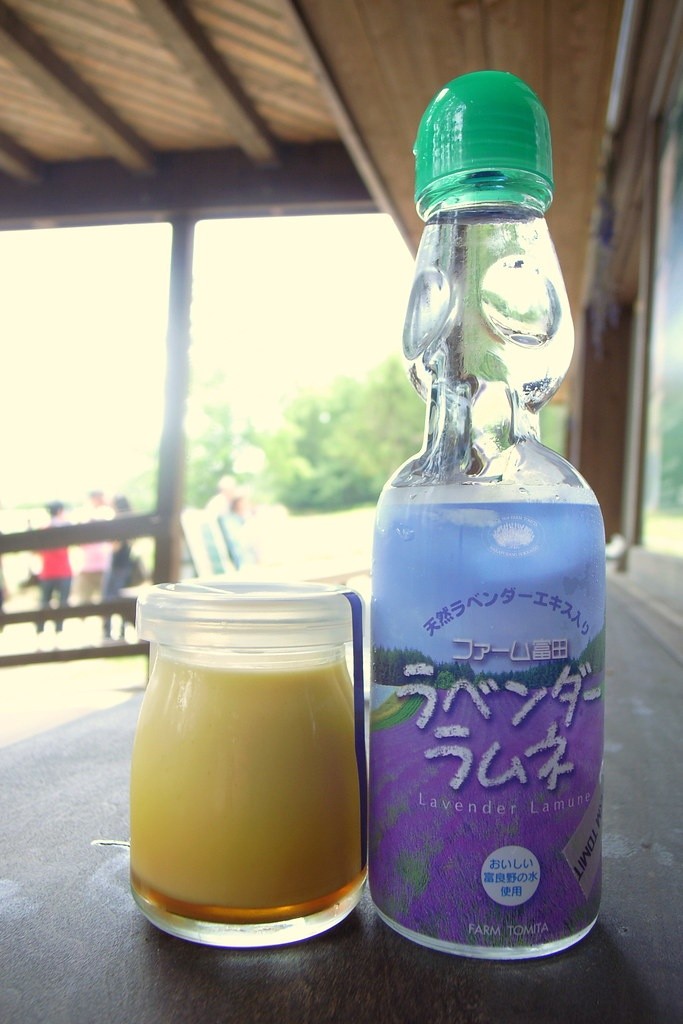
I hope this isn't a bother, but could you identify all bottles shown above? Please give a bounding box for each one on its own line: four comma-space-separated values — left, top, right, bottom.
368, 71, 603, 960
126, 581, 366, 947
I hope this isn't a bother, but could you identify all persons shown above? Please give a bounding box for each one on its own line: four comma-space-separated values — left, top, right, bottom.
35, 501, 75, 652
74, 477, 254, 646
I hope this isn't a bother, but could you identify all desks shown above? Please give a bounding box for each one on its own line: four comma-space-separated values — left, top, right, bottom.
0, 590, 683, 1024
